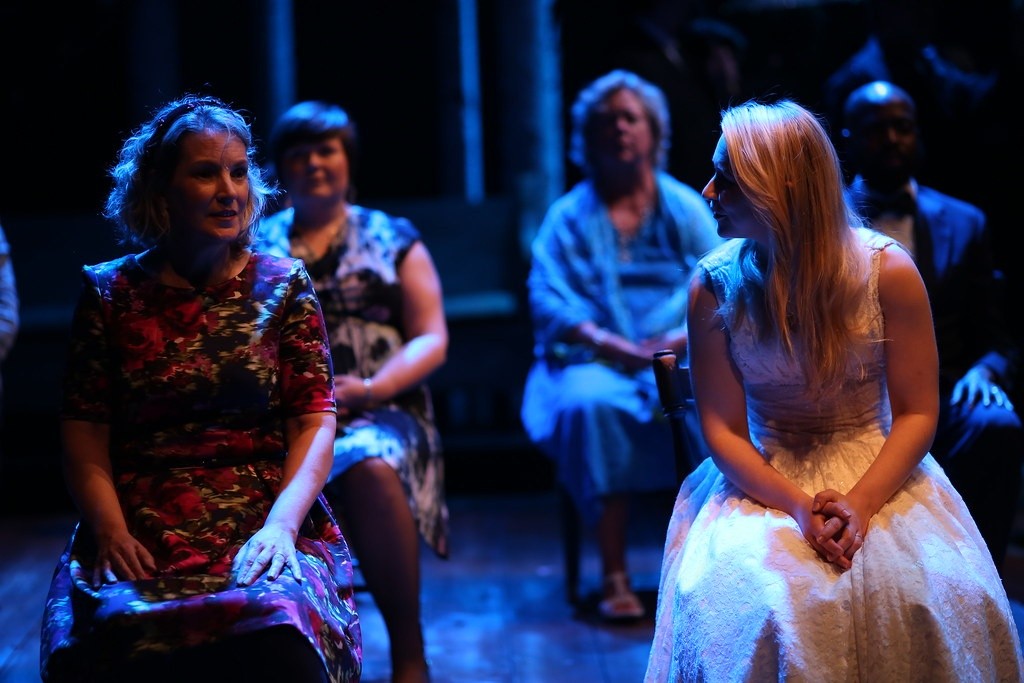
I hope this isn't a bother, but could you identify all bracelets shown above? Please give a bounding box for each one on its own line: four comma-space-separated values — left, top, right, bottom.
365, 378, 373, 401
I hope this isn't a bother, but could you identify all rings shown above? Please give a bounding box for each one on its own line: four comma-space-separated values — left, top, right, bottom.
855, 534, 864, 542
275, 552, 288, 560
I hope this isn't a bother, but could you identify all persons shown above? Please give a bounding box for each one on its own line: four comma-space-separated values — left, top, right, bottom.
822, 0, 1024, 182
39, 96, 364, 683
643, 100, 1024, 682
841, 82, 1024, 590
518, 68, 730, 624
250, 101, 451, 683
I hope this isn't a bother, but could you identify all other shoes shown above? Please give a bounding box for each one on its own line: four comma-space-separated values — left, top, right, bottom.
592, 568, 650, 625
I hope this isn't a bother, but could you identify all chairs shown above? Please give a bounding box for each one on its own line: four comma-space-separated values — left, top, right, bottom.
654, 347, 714, 485
521, 187, 626, 606
